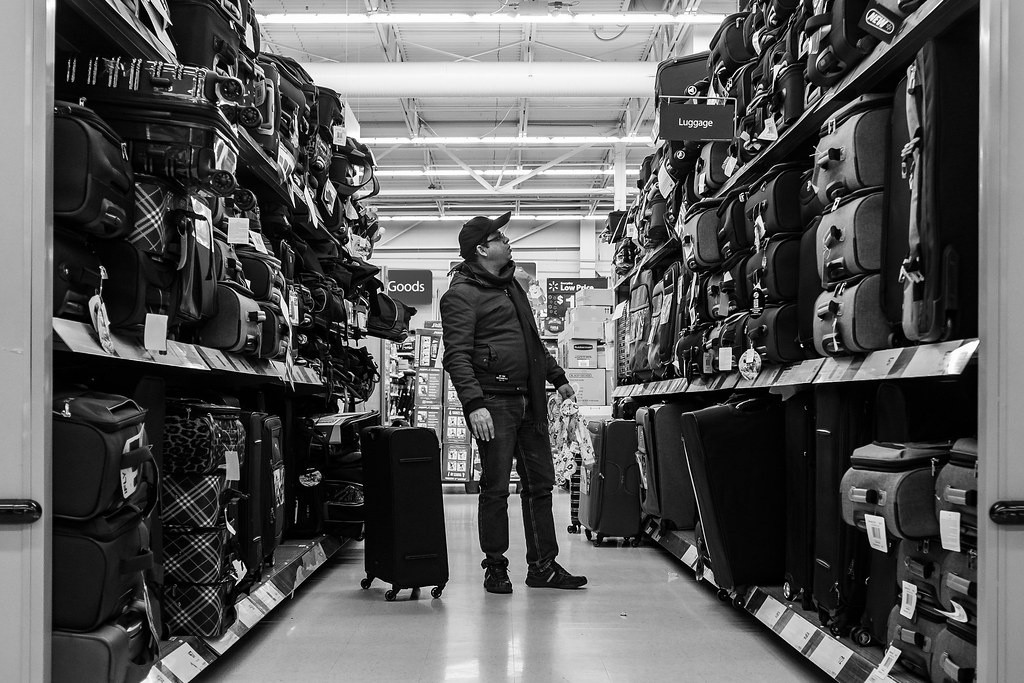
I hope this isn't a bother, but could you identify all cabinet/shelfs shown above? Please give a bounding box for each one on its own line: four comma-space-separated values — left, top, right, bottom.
46, 1, 358, 683
613, 0, 997, 683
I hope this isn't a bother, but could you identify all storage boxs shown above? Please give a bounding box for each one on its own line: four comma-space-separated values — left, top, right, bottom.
545, 285, 620, 407
414, 329, 483, 482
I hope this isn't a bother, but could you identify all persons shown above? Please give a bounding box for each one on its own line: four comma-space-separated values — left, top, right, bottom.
440, 210, 587, 594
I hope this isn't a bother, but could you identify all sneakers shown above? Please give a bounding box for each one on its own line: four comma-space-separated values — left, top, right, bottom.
484, 565, 512, 593
526, 562, 587, 589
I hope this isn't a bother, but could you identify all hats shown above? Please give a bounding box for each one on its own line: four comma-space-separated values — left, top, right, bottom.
460, 212, 511, 256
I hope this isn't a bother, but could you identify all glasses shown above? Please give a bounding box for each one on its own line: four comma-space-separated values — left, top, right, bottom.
482, 232, 502, 246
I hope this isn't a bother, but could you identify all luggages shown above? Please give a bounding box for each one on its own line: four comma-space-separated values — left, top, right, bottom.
361, 420, 448, 601
51, 0, 415, 683
580, 1, 977, 683
